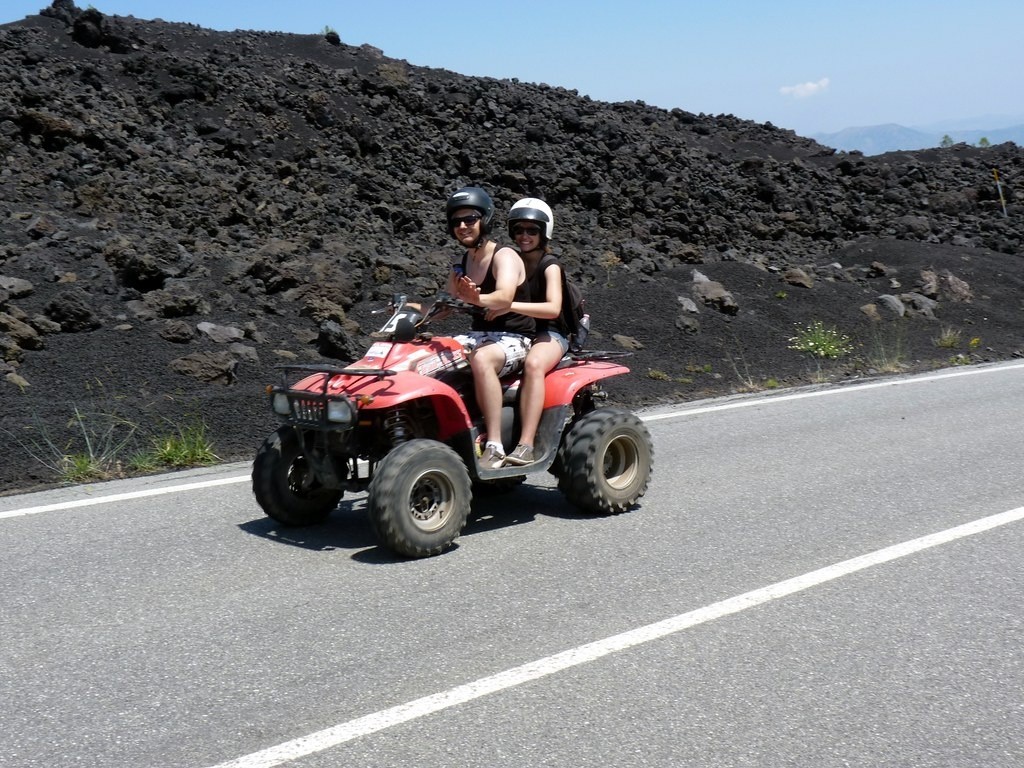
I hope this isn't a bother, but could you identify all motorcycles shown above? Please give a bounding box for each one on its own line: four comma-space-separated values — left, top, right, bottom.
249, 293, 654, 559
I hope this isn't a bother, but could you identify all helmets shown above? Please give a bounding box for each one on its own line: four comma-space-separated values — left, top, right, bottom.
508, 198, 554, 240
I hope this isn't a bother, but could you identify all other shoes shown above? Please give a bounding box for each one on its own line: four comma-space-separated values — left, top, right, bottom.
478, 448, 507, 469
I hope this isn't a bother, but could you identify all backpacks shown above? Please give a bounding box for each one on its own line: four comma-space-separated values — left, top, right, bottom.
537, 255, 590, 353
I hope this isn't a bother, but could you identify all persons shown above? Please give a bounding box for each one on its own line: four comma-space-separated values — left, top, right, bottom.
387, 187, 538, 470
448, 196, 573, 465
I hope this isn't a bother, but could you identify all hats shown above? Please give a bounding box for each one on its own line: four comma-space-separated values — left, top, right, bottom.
446, 188, 494, 240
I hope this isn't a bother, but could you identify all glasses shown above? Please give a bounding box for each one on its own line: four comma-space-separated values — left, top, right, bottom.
451, 215, 481, 228
511, 222, 541, 236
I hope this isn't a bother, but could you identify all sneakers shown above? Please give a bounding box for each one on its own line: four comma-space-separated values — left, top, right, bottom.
506, 445, 534, 464
